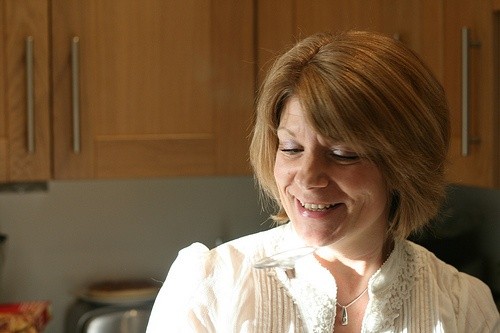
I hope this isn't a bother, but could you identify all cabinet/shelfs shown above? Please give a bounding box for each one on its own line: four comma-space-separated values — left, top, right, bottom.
0, 0, 500, 191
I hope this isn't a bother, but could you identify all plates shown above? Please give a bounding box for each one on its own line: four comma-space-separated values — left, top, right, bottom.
77, 279, 159, 306
